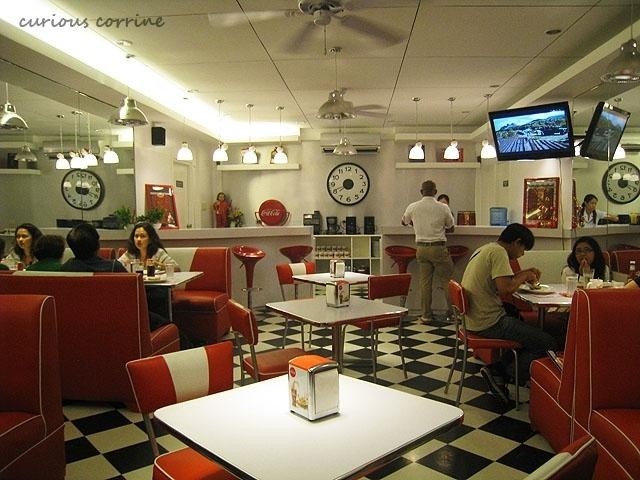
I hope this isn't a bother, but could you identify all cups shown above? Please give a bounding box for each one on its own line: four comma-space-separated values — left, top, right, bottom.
134, 259, 144, 279
583, 268, 594, 289
165, 261, 175, 280
566, 273, 578, 296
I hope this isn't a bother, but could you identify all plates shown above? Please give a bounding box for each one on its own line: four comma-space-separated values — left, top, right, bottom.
519, 284, 556, 294
143, 275, 167, 282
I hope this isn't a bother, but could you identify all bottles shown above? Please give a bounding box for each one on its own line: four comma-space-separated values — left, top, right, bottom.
147, 259, 155, 277
345, 260, 356, 272
627, 261, 639, 284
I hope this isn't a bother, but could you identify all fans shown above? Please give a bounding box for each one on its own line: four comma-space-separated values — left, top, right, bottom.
290, 88, 391, 119
207, 0, 409, 55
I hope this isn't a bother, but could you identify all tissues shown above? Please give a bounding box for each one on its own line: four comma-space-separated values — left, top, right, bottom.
288, 354, 340, 422
325, 280, 351, 308
330, 259, 345, 278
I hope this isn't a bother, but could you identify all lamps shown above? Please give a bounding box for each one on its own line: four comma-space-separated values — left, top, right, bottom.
55, 115, 70, 170
315, 51, 358, 155
105, 55, 149, 129
84, 113, 98, 167
600, 0, 639, 84
177, 98, 288, 164
70, 111, 82, 169
613, 97, 626, 159
409, 95, 497, 159
0, 80, 30, 132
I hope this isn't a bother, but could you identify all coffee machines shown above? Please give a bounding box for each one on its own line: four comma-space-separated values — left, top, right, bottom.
326, 216, 375, 235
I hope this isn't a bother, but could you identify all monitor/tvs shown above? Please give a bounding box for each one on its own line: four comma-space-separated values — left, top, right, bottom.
580, 101, 633, 162
487, 101, 576, 162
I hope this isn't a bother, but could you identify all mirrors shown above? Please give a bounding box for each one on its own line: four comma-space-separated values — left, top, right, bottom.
572, 83, 640, 250
0, 55, 138, 263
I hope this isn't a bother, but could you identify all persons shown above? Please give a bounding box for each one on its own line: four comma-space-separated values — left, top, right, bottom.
577, 194, 619, 227
460, 223, 559, 406
437, 194, 456, 219
26, 234, 65, 271
214, 192, 231, 228
2, 222, 43, 269
402, 181, 461, 324
560, 235, 610, 284
115, 222, 180, 329
0, 240, 10, 269
59, 222, 129, 273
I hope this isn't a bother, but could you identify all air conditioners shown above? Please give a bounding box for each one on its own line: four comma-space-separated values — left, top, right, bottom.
320, 133, 381, 155
43, 141, 100, 160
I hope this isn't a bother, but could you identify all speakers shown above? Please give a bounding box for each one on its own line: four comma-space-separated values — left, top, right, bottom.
151, 127, 166, 145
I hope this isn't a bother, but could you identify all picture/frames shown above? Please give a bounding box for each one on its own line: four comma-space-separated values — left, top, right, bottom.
145, 184, 180, 229
523, 177, 559, 229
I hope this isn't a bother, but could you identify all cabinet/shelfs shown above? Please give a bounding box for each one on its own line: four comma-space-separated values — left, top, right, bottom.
313, 235, 382, 275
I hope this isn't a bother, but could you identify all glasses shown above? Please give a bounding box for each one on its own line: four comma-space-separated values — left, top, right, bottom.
575, 248, 592, 255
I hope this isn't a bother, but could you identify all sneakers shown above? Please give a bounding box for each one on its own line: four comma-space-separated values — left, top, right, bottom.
480, 366, 512, 403
421, 315, 431, 322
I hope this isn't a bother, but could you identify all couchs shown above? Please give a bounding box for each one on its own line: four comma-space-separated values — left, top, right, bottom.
571, 289, 640, 480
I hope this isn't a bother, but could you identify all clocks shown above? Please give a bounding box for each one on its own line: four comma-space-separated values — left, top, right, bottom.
601, 162, 640, 205
61, 169, 104, 210
327, 162, 370, 205
